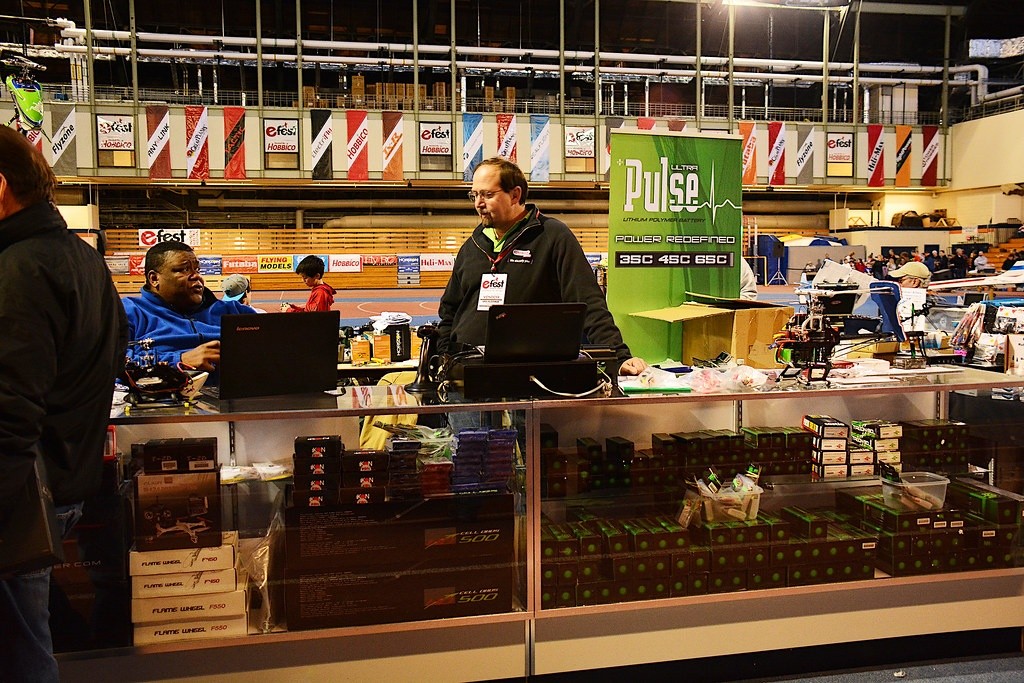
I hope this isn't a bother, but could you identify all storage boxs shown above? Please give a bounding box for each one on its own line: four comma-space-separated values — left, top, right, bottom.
50, 425, 518, 647
627, 289, 796, 368
844, 307, 1024, 374
538, 389, 1024, 608
341, 322, 422, 364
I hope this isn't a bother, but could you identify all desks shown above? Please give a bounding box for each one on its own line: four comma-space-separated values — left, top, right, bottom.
337, 358, 421, 369
534, 362, 1023, 682
47, 381, 534, 682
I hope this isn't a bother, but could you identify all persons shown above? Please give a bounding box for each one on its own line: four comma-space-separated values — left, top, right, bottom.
221, 272, 252, 307
740, 254, 757, 301
1002, 249, 1024, 270
888, 262, 948, 305
281, 255, 337, 312
0, 123, 129, 683
803, 249, 988, 282
436, 157, 647, 430
116, 241, 258, 386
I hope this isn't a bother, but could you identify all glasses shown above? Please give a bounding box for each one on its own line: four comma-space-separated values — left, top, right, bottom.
468, 185, 512, 203
898, 277, 914, 283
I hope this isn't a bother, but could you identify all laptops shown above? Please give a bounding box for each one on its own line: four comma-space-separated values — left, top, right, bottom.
479, 302, 588, 364
199, 310, 341, 400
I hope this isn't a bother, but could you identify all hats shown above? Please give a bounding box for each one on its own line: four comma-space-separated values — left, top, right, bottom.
979, 251, 983, 255
956, 248, 964, 253
888, 261, 930, 279
221, 274, 248, 301
